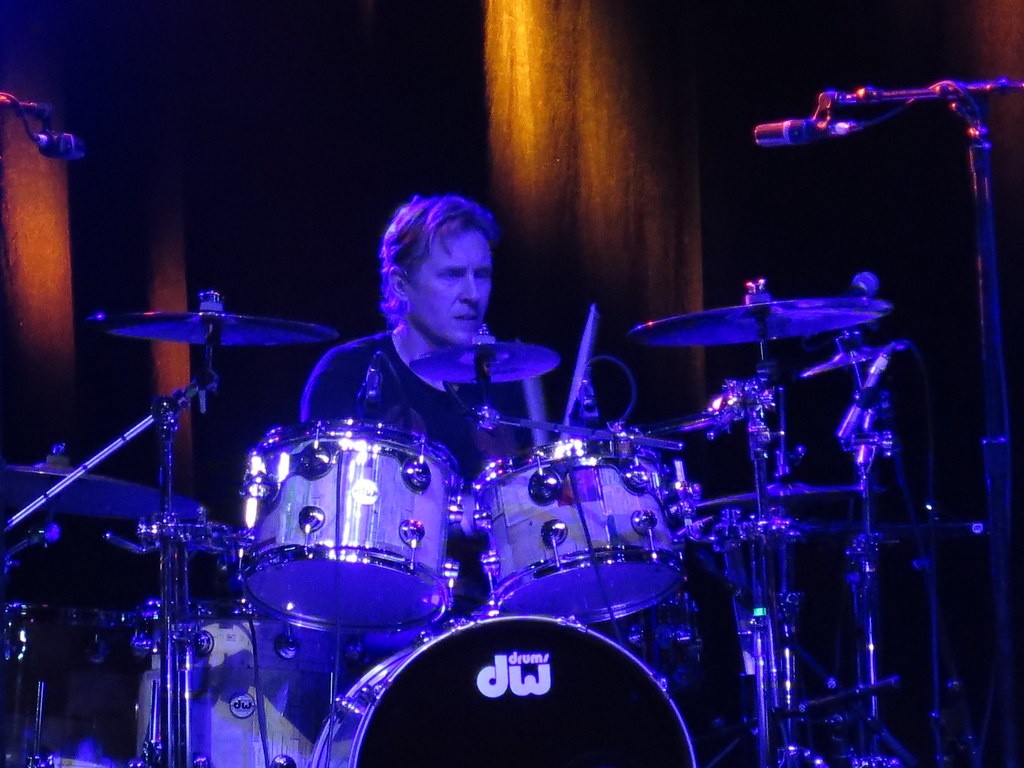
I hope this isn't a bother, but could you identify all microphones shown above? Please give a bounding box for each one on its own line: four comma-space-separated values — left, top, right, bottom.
363, 360, 386, 420
753, 119, 862, 148
803, 273, 881, 350
36, 131, 85, 159
839, 348, 893, 439
581, 369, 601, 431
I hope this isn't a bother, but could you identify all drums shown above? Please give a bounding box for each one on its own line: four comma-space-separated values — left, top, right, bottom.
131, 609, 343, 768
238, 417, 459, 625
472, 437, 686, 624
312, 614, 700, 767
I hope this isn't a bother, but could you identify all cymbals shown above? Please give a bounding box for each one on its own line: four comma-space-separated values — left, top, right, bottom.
0, 460, 210, 521
628, 297, 894, 347
100, 309, 339, 349
695, 481, 887, 508
798, 344, 885, 378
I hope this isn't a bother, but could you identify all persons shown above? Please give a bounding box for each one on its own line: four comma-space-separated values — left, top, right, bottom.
297, 194, 520, 491
